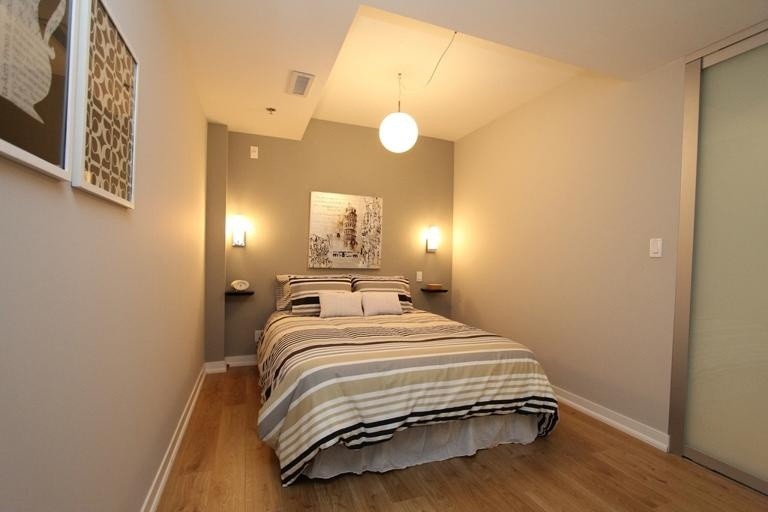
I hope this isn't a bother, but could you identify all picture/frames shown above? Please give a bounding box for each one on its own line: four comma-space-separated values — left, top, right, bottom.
72, 0, 141, 209
0, 0, 80, 182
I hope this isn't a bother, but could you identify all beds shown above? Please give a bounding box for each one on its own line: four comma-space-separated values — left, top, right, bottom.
256, 310, 560, 487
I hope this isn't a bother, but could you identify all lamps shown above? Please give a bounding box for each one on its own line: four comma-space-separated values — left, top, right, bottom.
229, 214, 246, 246
427, 227, 440, 253
379, 73, 419, 153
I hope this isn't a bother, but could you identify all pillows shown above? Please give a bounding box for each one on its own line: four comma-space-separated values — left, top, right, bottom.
276, 273, 414, 316
